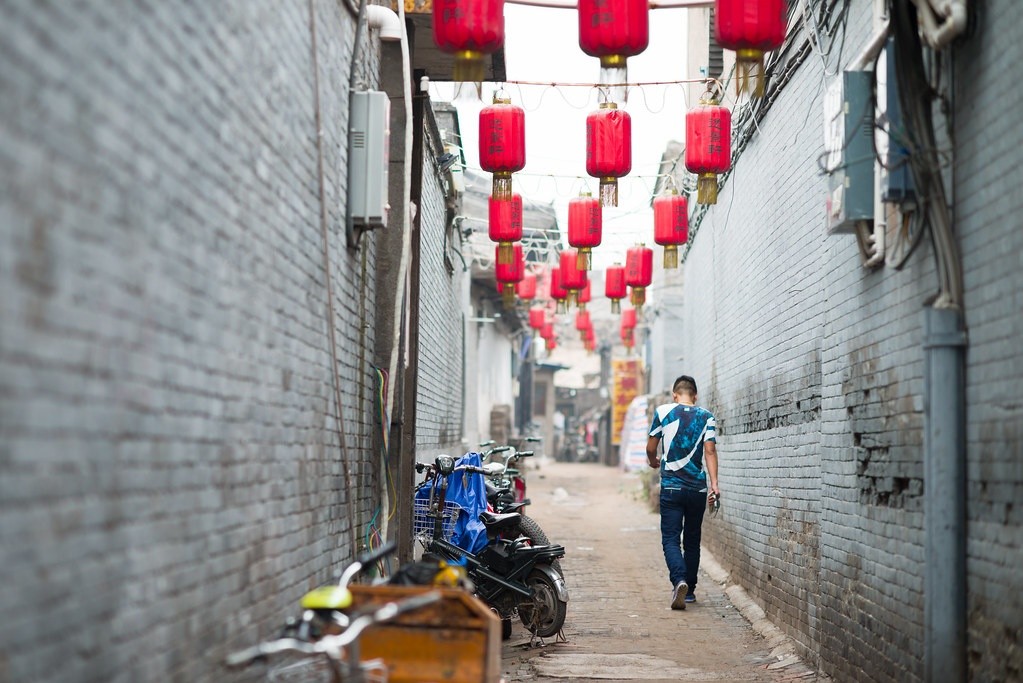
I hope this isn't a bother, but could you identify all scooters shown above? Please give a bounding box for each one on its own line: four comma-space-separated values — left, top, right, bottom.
412, 454, 569, 650
236, 539, 403, 683
479, 436, 542, 518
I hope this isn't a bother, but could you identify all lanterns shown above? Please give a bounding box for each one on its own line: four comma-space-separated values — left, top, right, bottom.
559, 248, 587, 307
578, 279, 592, 308
494, 242, 525, 306
495, 281, 518, 294
576, 1, 651, 69
550, 270, 567, 314
624, 240, 654, 309
517, 273, 537, 304
568, 178, 603, 271
653, 177, 688, 269
586, 86, 632, 208
485, 193, 523, 265
711, 0, 788, 97
432, 1, 506, 84
684, 83, 732, 206
478, 84, 526, 202
527, 301, 645, 358
606, 261, 627, 314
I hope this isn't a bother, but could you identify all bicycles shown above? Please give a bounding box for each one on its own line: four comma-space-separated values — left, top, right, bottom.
218, 587, 443, 683
451, 442, 566, 585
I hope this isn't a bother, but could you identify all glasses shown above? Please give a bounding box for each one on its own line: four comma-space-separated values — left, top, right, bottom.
712, 492, 720, 519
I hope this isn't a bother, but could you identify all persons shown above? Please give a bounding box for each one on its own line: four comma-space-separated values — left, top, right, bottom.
646, 375, 722, 610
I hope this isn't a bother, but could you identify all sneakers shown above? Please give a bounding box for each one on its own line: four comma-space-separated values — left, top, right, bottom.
671, 580, 689, 611
685, 594, 696, 602
